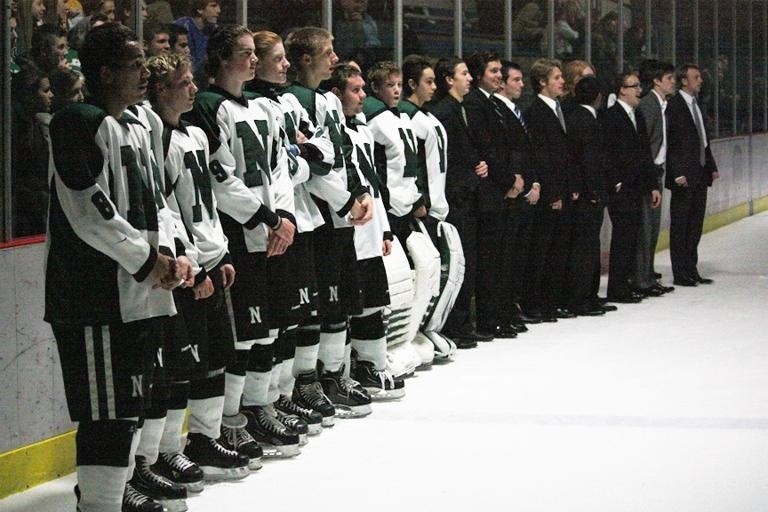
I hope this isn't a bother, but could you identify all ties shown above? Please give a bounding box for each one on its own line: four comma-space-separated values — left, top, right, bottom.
514, 105, 527, 133
691, 98, 706, 168
555, 102, 567, 135
489, 94, 503, 129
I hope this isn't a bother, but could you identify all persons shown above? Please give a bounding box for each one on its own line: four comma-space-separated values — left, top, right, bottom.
144, 51, 265, 469
182, 23, 308, 445
283, 24, 404, 406
400, 53, 458, 358
323, 65, 415, 379
243, 28, 337, 424
361, 60, 436, 367
40, 19, 181, 512
428, 49, 718, 349
304, 1, 767, 137
1, 1, 225, 243
125, 102, 205, 498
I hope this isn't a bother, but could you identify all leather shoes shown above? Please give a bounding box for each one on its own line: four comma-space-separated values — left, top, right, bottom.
452, 272, 713, 348
